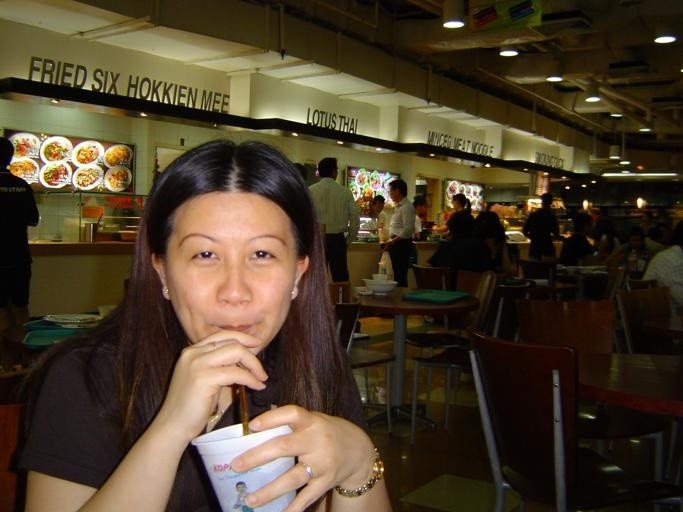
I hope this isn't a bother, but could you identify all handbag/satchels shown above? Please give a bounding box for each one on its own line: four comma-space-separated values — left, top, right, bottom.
408, 241, 417, 269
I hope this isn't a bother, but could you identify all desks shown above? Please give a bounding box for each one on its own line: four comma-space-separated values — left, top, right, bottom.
568, 336, 682, 483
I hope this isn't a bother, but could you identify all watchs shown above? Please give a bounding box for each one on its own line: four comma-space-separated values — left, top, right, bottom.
331, 450, 388, 496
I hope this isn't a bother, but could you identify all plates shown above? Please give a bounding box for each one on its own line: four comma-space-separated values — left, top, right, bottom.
4, 131, 134, 195
446, 180, 484, 211
353, 286, 373, 296
43, 313, 104, 328
344, 170, 400, 203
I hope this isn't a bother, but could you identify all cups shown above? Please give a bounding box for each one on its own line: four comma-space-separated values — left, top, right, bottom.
371, 274, 387, 279
186, 419, 301, 512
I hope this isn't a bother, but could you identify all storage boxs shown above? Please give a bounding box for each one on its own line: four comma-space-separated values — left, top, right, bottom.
414, 179, 427, 193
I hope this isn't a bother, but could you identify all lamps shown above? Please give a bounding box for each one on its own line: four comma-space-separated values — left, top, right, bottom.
619, 149, 633, 165
607, 141, 624, 160
439, 0, 682, 135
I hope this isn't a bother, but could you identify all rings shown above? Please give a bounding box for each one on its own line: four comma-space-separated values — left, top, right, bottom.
296, 457, 318, 482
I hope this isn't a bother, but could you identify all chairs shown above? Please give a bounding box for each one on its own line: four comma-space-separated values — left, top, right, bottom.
513, 297, 669, 512
1, 367, 51, 512
463, 325, 682, 512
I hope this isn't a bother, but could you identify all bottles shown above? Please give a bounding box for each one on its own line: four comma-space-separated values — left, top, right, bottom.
626, 249, 646, 272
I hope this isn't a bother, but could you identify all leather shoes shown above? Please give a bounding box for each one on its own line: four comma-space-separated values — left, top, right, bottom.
353, 333, 369, 338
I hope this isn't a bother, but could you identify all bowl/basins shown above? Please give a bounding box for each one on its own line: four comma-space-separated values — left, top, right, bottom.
362, 278, 398, 296
97, 304, 118, 317
561, 265, 606, 274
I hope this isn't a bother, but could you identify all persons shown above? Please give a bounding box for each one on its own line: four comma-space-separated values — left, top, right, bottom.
295, 147, 683, 357
14, 139, 397, 510
0, 134, 37, 325
